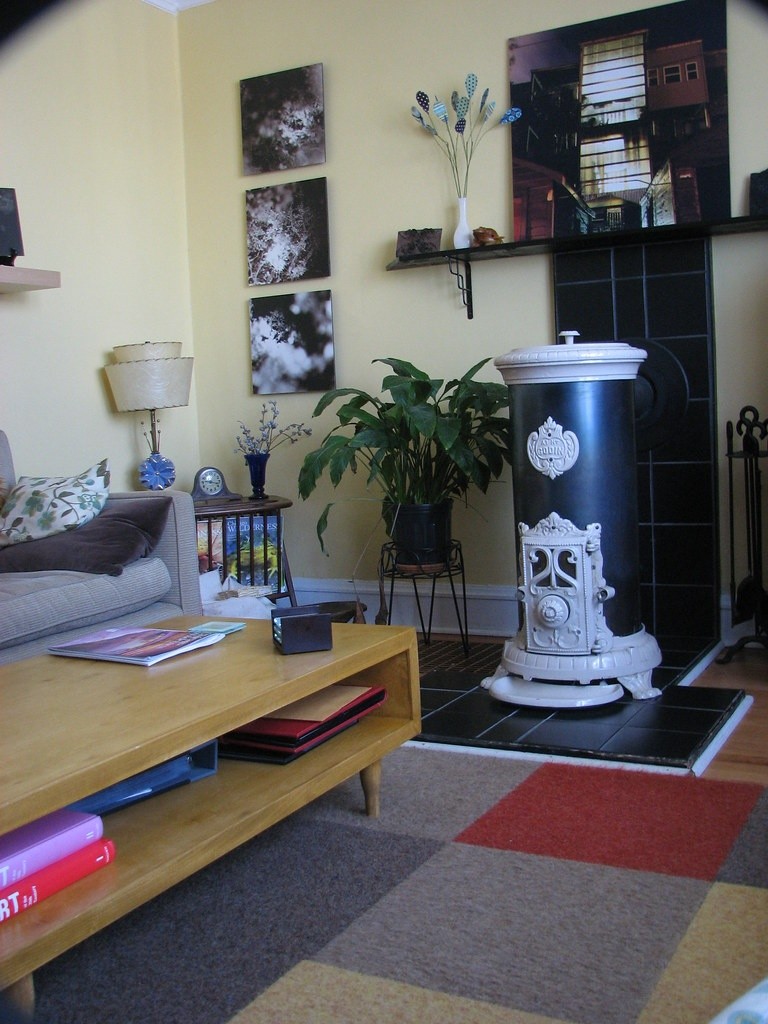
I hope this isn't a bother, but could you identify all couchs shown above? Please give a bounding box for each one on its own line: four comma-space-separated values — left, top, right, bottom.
0, 430, 202, 673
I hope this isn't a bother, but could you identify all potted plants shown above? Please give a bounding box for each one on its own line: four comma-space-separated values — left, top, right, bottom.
298, 358, 511, 569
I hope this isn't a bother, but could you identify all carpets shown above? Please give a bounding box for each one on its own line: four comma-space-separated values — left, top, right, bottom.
0, 741, 768, 1024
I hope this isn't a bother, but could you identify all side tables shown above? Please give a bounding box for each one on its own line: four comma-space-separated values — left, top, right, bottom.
195, 496, 298, 607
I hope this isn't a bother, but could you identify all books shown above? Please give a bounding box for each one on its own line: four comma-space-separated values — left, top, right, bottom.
48, 625, 226, 667
65, 738, 218, 818
218, 683, 387, 765
197, 515, 288, 598
0, 809, 116, 924
188, 622, 246, 635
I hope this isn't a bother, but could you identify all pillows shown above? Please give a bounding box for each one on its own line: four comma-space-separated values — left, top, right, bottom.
0, 457, 110, 547
0, 493, 173, 576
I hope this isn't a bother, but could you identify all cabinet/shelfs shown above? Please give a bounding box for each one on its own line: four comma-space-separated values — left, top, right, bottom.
719, 405, 768, 666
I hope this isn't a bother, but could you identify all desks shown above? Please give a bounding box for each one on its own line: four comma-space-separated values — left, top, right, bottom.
376, 542, 469, 656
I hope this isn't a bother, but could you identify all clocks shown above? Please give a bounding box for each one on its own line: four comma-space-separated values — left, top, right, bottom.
192, 466, 242, 500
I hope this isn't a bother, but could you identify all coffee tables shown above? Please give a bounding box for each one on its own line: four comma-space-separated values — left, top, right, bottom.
0, 613, 423, 1019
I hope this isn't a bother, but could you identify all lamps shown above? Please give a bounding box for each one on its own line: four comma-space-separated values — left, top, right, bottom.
105, 340, 195, 489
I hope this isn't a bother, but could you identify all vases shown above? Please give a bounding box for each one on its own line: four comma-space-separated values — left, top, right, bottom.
453, 195, 474, 247
243, 454, 269, 499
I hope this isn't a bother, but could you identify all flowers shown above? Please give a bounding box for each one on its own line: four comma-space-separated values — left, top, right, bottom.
233, 400, 310, 454
